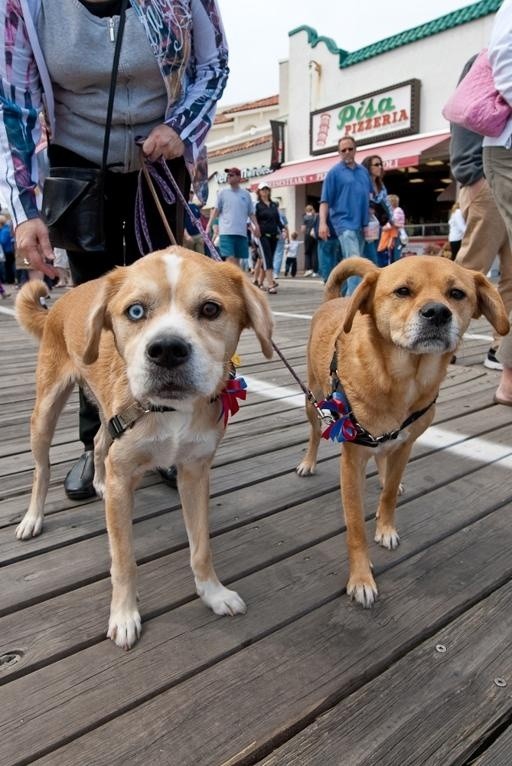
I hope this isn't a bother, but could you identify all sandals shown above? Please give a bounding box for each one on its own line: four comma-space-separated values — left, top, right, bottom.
493, 390, 512, 410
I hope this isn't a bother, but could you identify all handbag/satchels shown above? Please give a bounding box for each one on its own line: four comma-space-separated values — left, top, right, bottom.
362, 219, 399, 253
33, 170, 113, 257
440, 46, 511, 139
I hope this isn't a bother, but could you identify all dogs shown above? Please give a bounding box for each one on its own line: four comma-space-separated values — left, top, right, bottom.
295, 253, 510, 610
10, 243, 275, 652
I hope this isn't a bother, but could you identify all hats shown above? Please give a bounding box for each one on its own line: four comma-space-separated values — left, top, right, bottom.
223, 166, 242, 177
257, 182, 271, 189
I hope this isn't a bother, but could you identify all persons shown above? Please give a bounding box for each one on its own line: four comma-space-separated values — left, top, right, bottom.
2, 0, 512, 498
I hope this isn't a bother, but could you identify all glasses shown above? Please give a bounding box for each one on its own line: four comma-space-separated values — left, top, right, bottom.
340, 147, 355, 155
226, 173, 238, 177
370, 163, 383, 167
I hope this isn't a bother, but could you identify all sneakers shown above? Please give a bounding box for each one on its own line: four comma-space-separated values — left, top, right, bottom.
251, 270, 280, 298
303, 269, 318, 280
482, 347, 507, 371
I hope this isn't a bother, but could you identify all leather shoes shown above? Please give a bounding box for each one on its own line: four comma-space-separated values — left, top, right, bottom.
156, 461, 179, 491
61, 447, 99, 500
